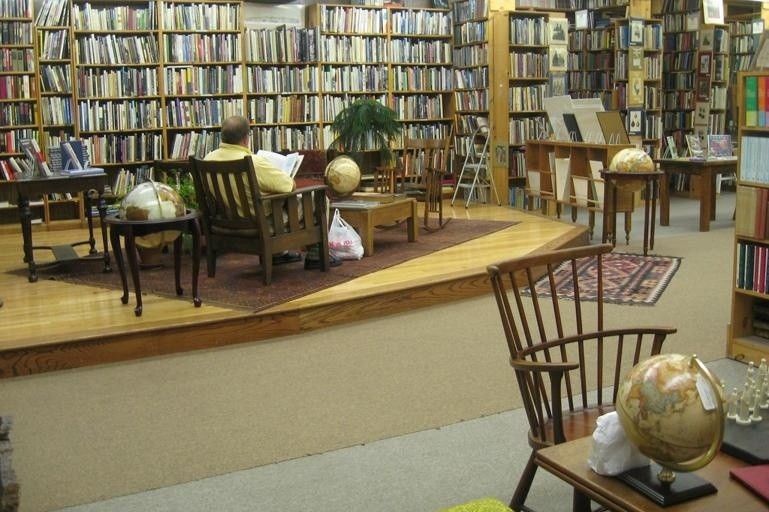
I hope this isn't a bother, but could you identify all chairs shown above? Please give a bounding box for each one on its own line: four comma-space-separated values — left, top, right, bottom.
373, 135, 452, 233
189, 155, 330, 286
486, 243, 677, 511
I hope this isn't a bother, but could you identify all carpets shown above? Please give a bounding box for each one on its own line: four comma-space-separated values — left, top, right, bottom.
1, 228, 735, 511
6, 217, 524, 314
519, 252, 685, 306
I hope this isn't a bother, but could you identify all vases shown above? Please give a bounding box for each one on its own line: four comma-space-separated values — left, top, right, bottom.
137, 245, 161, 266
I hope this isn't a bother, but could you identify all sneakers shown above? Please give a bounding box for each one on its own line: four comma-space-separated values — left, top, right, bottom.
272, 251, 301, 265
304, 254, 341, 270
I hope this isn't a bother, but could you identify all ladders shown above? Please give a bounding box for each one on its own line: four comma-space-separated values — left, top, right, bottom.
449, 125, 502, 209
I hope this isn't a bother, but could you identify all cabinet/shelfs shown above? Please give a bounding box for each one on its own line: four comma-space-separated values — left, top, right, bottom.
726, 70, 769, 368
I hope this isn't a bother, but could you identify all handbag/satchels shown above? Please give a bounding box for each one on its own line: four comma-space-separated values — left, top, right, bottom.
589, 411, 650, 476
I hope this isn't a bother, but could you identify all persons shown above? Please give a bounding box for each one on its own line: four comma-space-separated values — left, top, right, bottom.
200, 114, 342, 272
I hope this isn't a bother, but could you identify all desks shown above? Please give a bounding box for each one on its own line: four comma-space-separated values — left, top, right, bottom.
330, 195, 419, 257
534, 436, 768, 512
2, 173, 113, 283
653, 158, 736, 232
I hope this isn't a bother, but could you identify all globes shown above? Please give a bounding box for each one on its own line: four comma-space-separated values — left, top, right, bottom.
614, 351, 731, 508
101, 177, 207, 317
322, 154, 362, 203
598, 147, 667, 257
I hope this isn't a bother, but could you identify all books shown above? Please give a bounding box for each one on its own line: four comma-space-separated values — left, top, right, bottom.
735, 216, 768, 349
0, 0, 768, 216
